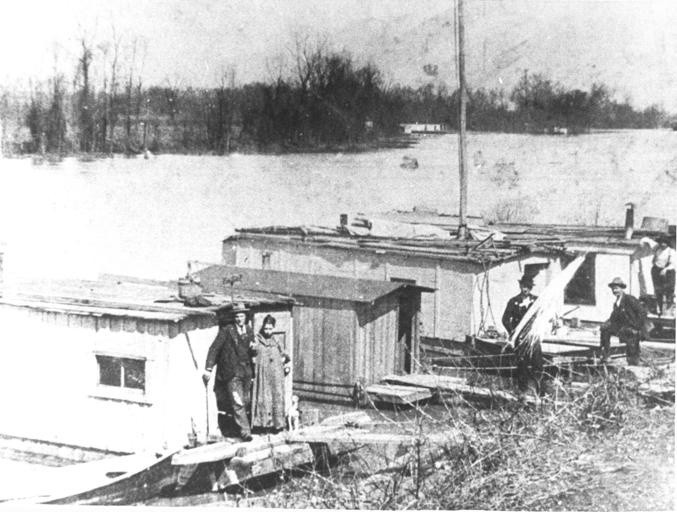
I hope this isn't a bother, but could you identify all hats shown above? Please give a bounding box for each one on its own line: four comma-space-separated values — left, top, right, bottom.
230, 301, 251, 312
517, 274, 535, 286
654, 235, 672, 245
607, 276, 627, 289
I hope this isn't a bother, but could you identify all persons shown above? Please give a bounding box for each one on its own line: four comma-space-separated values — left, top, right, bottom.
502, 274, 545, 394
249, 315, 290, 430
642, 235, 676, 315
598, 278, 650, 366
202, 301, 259, 442
